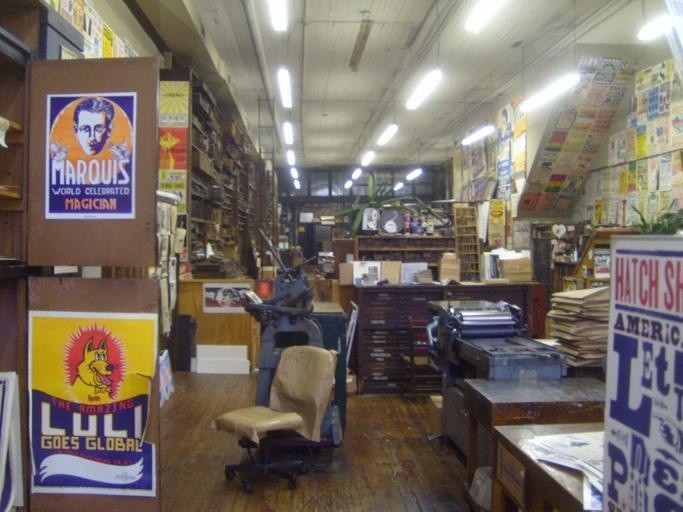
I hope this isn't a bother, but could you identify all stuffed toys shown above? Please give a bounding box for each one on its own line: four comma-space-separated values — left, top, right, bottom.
402, 213, 434, 236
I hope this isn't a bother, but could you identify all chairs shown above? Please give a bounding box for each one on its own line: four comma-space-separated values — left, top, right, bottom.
216, 343, 336, 494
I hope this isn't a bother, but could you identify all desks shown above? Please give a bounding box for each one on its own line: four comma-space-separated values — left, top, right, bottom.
174, 276, 263, 371
272, 301, 348, 431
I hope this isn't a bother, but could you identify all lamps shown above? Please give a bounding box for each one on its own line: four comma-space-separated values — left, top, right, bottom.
636, 16, 683, 43
376, 123, 399, 145
461, 125, 494, 146
282, 122, 295, 147
269, 0, 288, 33
277, 64, 293, 109
406, 167, 422, 181
518, 73, 583, 111
405, 69, 442, 111
360, 149, 375, 167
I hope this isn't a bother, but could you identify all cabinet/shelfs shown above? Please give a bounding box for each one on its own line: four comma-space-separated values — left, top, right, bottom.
342, 285, 549, 396
0, 29, 159, 272
0, 273, 160, 512
440, 337, 565, 452
162, 66, 279, 280
491, 422, 606, 512
44, 3, 84, 59
467, 375, 607, 463
455, 205, 480, 282
354, 234, 455, 280
0, 0, 43, 58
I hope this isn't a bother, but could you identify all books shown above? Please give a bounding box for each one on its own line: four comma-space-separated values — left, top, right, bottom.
190, 80, 275, 280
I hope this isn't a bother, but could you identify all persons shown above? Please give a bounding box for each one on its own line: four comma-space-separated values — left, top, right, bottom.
73, 98, 114, 156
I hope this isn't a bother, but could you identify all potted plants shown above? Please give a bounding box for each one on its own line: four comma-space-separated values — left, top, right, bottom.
334, 169, 447, 239
